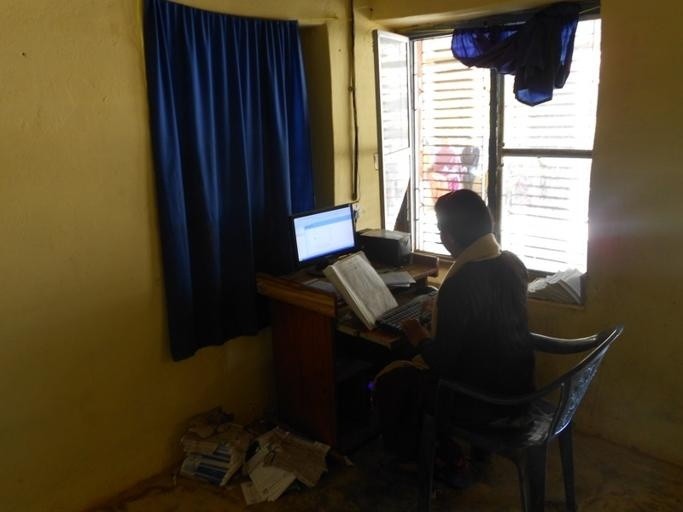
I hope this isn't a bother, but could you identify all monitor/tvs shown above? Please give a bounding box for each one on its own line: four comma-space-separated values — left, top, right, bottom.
290, 204, 356, 279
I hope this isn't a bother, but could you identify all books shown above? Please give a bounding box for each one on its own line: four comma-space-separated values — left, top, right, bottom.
324, 251, 399, 329
527, 269, 587, 306
176, 426, 254, 487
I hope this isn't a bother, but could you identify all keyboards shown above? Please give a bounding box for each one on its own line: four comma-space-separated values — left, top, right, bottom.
375, 293, 435, 335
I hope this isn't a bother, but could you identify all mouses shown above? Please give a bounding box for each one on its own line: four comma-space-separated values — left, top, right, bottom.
416, 285, 438, 296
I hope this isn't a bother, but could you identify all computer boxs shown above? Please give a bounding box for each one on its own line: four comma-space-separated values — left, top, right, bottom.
333, 351, 374, 434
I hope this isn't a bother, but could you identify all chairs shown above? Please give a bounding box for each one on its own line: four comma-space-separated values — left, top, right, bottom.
413, 327, 624, 512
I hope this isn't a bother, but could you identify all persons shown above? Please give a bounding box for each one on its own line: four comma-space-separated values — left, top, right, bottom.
330, 190, 534, 471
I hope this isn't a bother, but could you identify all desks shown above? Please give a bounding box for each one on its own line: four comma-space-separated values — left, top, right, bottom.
252, 251, 440, 462
